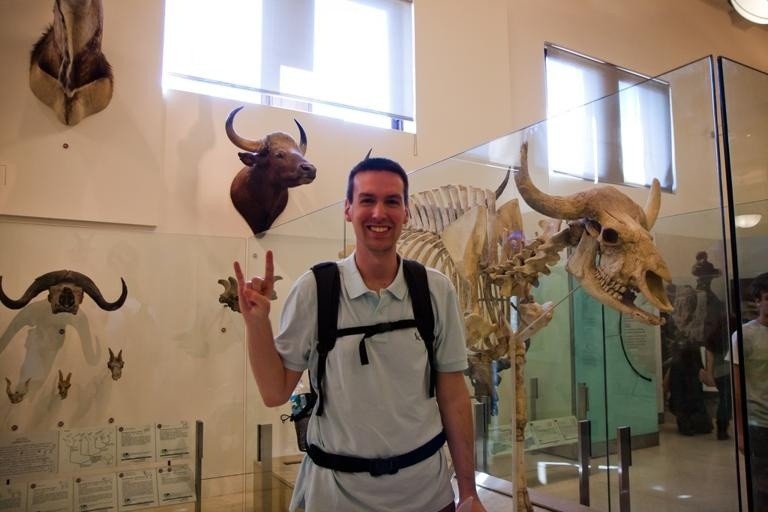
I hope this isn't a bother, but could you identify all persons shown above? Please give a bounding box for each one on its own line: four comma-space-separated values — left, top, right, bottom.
661, 311, 731, 440
724, 272, 768, 512
233, 158, 486, 512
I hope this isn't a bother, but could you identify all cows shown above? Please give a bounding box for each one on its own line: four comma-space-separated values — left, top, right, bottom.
225, 106, 317, 240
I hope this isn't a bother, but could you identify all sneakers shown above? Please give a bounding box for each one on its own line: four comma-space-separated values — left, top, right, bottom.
717, 433, 729, 440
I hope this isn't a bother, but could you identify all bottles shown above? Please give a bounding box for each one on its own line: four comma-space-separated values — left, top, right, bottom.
288, 377, 312, 451
